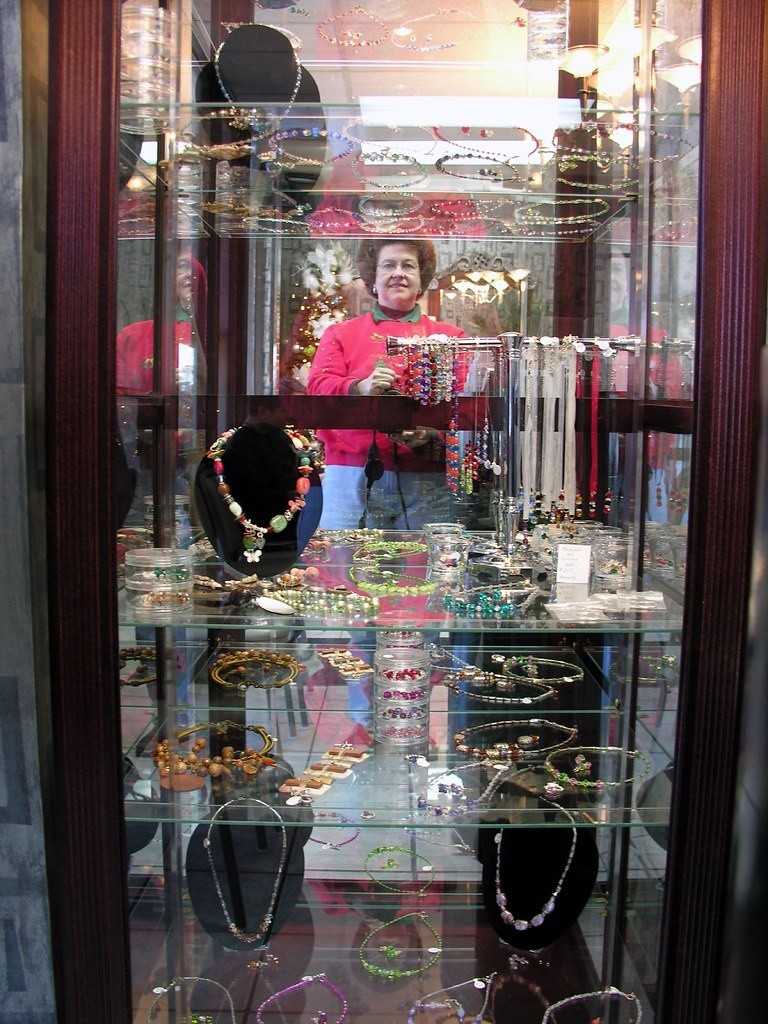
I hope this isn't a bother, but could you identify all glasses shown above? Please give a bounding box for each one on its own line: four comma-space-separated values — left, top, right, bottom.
376, 259, 420, 275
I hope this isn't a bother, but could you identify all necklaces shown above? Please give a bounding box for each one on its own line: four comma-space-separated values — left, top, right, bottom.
285, 420, 324, 474
153, 720, 654, 819
302, 520, 687, 567
204, 797, 289, 946
117, 493, 192, 551
610, 650, 680, 688
117, 564, 686, 632
631, 345, 692, 518
218, 2, 530, 53
493, 797, 576, 934
209, 426, 309, 566
211, 637, 589, 706
120, 647, 176, 686
213, 30, 301, 140
157, 104, 692, 194
365, 847, 438, 900
398, 335, 617, 524
303, 808, 360, 851
174, 191, 613, 242
146, 910, 646, 1024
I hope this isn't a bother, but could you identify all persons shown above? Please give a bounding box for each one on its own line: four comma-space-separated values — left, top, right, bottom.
307, 227, 486, 763
114, 243, 209, 729
